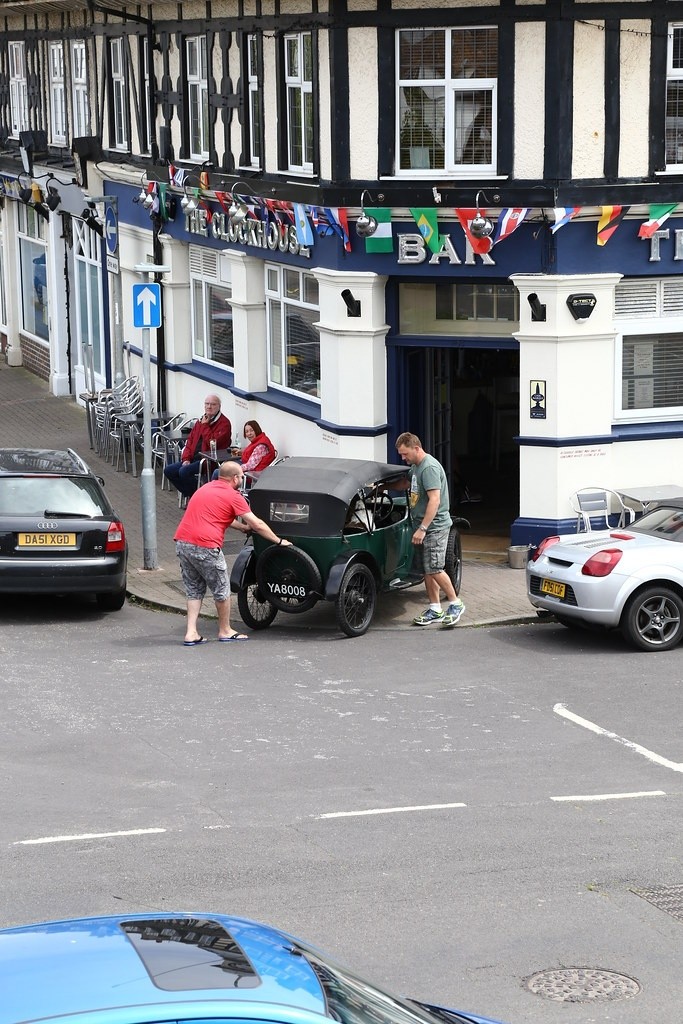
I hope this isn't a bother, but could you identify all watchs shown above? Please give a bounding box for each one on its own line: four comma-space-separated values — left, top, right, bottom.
419, 525, 427, 531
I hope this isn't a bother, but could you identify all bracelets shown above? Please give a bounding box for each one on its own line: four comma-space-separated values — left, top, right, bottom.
277, 539, 282, 545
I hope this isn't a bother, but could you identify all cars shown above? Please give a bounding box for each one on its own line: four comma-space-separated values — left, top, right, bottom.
527, 502, 683, 652
1, 447, 128, 612
1, 911, 505, 1023
231, 454, 470, 639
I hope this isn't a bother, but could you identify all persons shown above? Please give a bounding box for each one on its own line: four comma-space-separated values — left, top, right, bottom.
362, 432, 466, 625
164, 395, 231, 510
173, 461, 293, 646
210, 421, 275, 481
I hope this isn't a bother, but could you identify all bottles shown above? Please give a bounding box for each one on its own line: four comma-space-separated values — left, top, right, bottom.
235, 433, 241, 448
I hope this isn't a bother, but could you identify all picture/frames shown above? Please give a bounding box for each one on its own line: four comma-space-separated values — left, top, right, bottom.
529, 379, 546, 419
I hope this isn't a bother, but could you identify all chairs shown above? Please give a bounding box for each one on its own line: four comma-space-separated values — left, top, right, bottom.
90, 375, 309, 523
568, 486, 635, 536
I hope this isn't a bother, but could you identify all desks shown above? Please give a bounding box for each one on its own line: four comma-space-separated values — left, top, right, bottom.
198, 448, 241, 462
156, 430, 191, 463
114, 411, 182, 478
78, 392, 101, 449
614, 483, 683, 515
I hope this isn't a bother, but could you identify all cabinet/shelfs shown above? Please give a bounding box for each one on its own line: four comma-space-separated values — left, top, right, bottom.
488, 375, 520, 470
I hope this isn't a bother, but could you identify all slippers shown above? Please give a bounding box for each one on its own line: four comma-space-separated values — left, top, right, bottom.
184, 636, 209, 646
219, 632, 250, 642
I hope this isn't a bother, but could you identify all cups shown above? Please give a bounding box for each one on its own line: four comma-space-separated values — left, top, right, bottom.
210, 439, 216, 454
231, 448, 238, 457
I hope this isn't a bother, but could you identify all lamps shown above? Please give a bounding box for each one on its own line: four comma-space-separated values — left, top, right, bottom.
228, 181, 277, 226
80, 209, 90, 219
139, 170, 175, 210
45, 178, 77, 211
470, 190, 501, 239
355, 189, 385, 238
17, 171, 54, 203
180, 174, 226, 217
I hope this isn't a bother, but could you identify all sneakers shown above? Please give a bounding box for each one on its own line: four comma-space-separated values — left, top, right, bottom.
413, 607, 445, 625
442, 598, 465, 625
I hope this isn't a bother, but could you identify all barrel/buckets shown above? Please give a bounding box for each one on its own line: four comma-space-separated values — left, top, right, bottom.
506, 544, 537, 568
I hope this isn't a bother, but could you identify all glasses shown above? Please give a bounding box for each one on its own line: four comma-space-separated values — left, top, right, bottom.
234, 474, 244, 479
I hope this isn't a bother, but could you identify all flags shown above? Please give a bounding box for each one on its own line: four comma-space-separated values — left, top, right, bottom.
148, 166, 351, 251
597, 205, 632, 246
455, 208, 489, 253
491, 208, 531, 245
410, 208, 445, 254
364, 208, 393, 252
638, 202, 678, 237
551, 207, 579, 234
